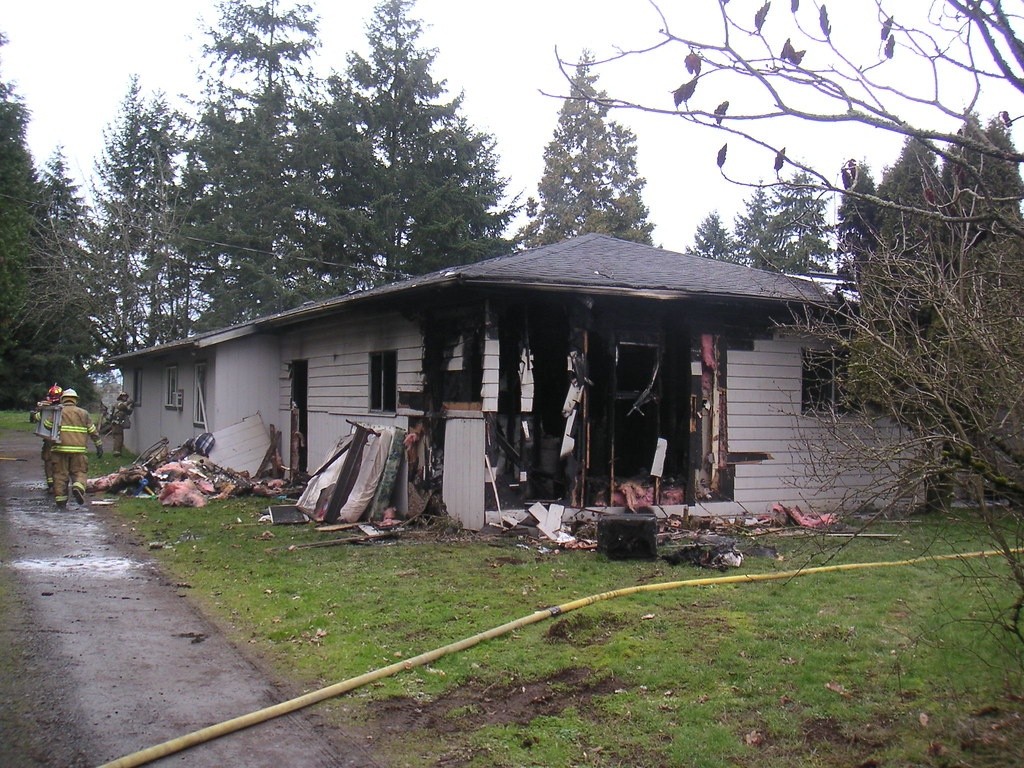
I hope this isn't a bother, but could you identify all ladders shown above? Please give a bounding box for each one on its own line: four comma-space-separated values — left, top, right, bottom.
29, 404, 63, 444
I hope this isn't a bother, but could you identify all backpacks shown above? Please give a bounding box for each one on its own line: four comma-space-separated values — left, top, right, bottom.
105, 403, 124, 424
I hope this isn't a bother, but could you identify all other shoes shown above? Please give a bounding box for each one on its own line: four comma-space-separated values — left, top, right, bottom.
48, 487, 54, 494
56, 502, 66, 509
72, 487, 84, 505
113, 453, 122, 457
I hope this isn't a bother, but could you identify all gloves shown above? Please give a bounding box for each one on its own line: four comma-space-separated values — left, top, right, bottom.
96, 445, 103, 459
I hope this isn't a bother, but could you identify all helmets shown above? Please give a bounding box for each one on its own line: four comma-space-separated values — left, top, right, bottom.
49, 383, 62, 399
60, 388, 79, 397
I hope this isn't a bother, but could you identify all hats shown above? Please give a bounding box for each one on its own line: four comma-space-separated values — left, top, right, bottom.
117, 391, 129, 399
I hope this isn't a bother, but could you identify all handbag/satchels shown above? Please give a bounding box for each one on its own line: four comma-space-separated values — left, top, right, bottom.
119, 418, 131, 429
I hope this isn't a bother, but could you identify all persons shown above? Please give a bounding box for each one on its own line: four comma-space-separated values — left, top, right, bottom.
31, 384, 64, 494
109, 391, 137, 458
44, 388, 103, 512
